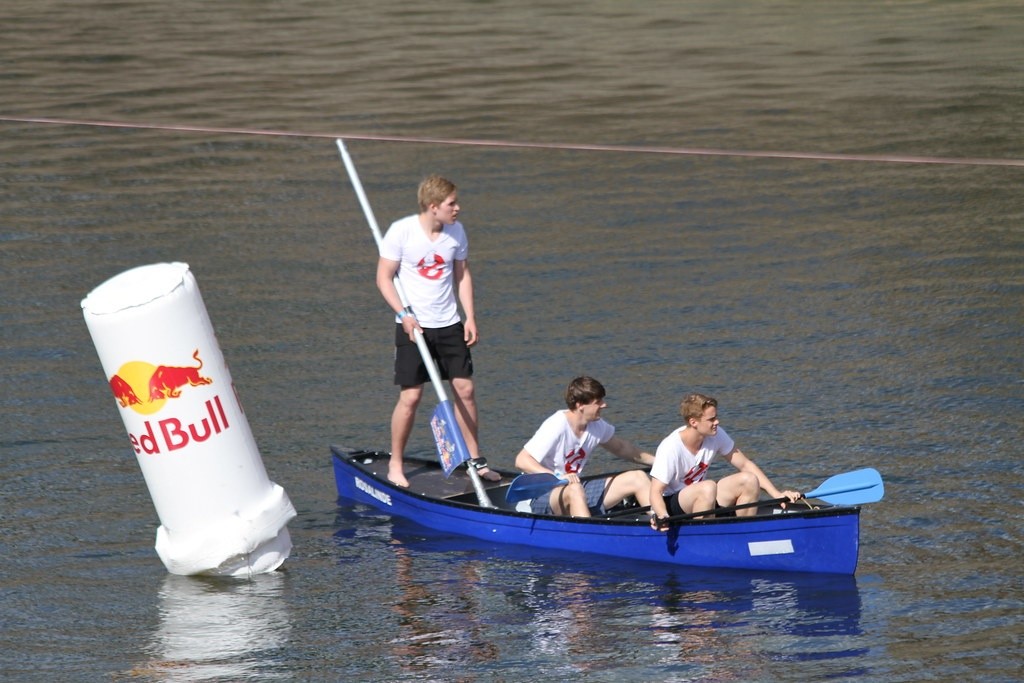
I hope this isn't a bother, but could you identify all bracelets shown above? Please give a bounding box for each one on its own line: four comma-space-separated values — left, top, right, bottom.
555, 473, 560, 478
398, 308, 406, 318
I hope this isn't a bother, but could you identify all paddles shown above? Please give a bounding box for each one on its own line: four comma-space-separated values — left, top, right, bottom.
505, 465, 650, 504
334, 137, 497, 507
652, 468, 885, 532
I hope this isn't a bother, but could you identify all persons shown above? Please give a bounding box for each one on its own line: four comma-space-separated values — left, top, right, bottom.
514, 376, 655, 517
373, 176, 501, 488
648, 393, 801, 532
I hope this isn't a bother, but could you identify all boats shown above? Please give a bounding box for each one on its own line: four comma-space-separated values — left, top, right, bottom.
329, 439, 863, 580
